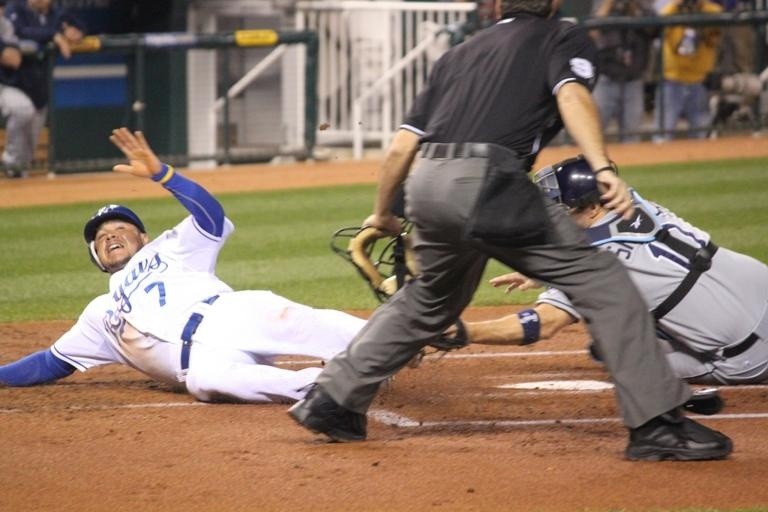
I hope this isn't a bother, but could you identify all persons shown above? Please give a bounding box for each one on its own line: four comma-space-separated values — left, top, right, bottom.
288, 3, 733, 462
1, 128, 427, 405
1, 0, 103, 181
382, 154, 766, 389
580, 1, 767, 143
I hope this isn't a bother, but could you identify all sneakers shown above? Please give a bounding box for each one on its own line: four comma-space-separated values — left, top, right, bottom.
627, 412, 733, 461
285, 383, 369, 442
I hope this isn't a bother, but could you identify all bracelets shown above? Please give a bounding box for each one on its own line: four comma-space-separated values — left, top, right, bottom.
154, 166, 178, 184
518, 309, 540, 347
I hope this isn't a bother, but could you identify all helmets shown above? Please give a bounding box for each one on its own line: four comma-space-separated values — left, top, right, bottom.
83, 203, 146, 242
531, 151, 618, 211
